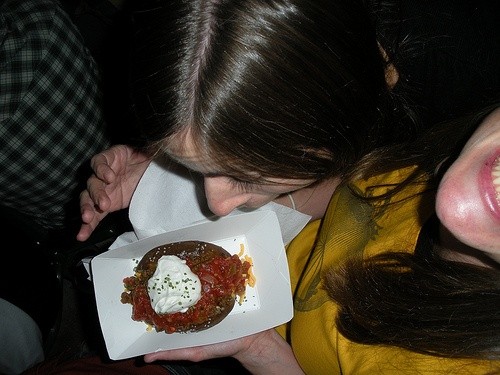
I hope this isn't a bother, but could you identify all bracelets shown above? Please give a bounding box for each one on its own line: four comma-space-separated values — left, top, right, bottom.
286, 193, 297, 212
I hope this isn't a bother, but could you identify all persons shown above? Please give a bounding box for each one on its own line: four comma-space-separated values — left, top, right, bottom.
17, 333, 228, 375
1, 0, 106, 235
76, 107, 500, 375
107, 0, 500, 217
